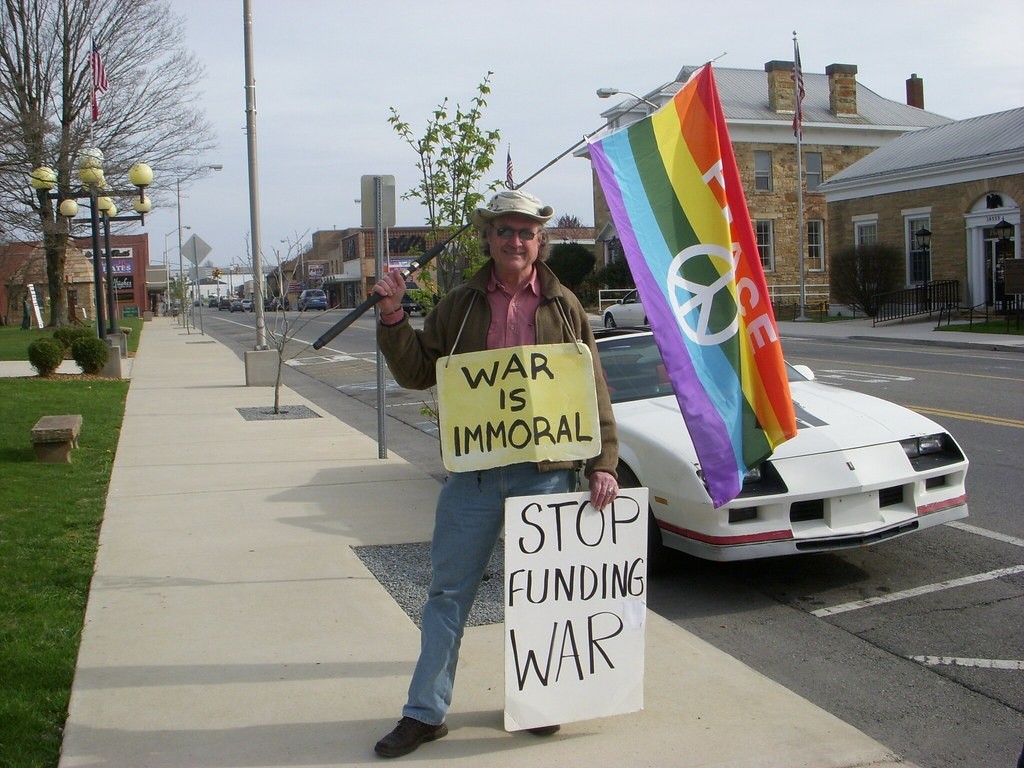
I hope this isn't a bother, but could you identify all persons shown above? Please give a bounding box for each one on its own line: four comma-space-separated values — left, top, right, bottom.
152, 296, 159, 317
250, 296, 255, 312
20, 291, 32, 330
374, 191, 620, 757
269, 296, 276, 311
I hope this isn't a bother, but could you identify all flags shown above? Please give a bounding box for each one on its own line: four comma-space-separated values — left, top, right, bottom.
588, 62, 797, 510
507, 152, 513, 183
791, 45, 805, 139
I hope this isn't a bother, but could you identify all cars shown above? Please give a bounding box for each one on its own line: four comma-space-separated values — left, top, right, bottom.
193, 292, 290, 313
296, 289, 328, 311
586, 329, 971, 576
400, 281, 427, 318
601, 285, 656, 328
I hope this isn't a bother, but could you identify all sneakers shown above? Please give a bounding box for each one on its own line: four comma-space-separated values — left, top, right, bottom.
374, 716, 448, 757
526, 725, 560, 736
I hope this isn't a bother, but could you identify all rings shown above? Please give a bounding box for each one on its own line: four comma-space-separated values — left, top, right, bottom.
608, 487, 613, 494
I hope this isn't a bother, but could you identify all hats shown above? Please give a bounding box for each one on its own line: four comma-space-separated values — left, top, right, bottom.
470, 190, 554, 228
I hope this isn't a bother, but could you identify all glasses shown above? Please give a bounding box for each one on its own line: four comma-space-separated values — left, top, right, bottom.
488, 222, 541, 241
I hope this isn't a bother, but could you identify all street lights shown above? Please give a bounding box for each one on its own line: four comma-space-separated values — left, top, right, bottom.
281, 238, 304, 281
996, 215, 1014, 313
163, 225, 192, 315
30, 147, 154, 340
175, 163, 223, 328
914, 225, 932, 313
59, 194, 152, 333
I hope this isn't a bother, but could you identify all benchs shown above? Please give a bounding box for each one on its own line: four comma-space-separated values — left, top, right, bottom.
29, 414, 82, 464
608, 374, 655, 391
